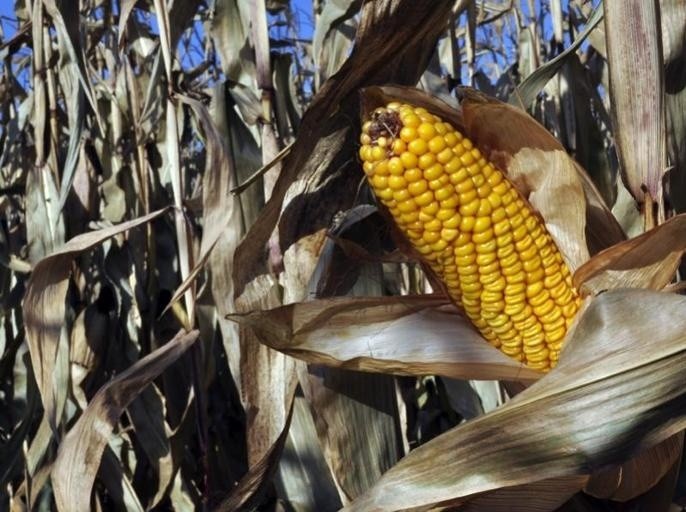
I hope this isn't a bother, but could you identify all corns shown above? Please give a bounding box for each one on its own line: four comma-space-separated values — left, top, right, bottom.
359, 102, 581, 374
